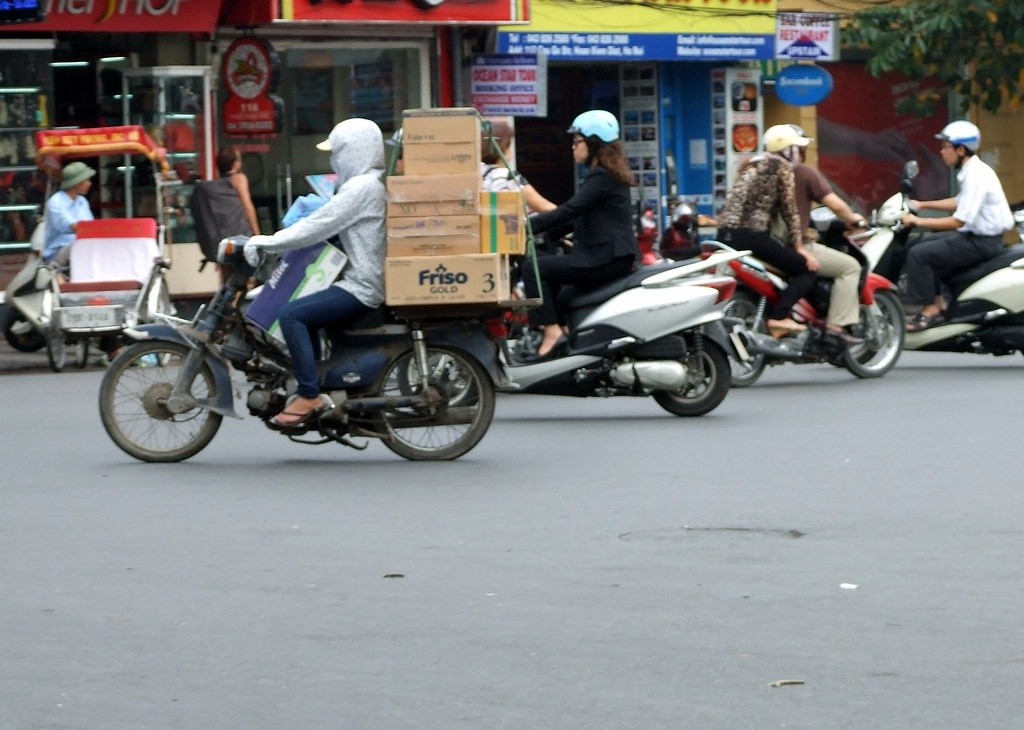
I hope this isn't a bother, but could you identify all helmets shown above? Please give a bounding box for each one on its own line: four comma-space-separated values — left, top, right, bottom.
763, 125, 809, 152
567, 110, 620, 143
672, 203, 692, 222
934, 120, 981, 150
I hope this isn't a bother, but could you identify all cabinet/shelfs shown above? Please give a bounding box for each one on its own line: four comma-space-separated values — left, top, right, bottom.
0, 54, 210, 249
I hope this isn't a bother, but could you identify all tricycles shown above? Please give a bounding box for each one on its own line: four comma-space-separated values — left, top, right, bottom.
35, 124, 176, 372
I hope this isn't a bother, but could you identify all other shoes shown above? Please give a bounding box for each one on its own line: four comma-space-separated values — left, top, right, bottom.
767, 320, 809, 338
821, 330, 863, 347
101, 355, 112, 367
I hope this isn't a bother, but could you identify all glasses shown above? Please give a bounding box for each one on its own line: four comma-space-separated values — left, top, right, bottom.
941, 142, 953, 149
573, 138, 585, 144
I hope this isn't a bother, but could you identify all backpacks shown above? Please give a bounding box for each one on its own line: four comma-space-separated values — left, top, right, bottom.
190, 177, 254, 262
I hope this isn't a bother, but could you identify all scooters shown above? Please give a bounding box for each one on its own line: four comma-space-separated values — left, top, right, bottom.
0, 214, 54, 352
98, 235, 542, 463
400, 156, 1024, 415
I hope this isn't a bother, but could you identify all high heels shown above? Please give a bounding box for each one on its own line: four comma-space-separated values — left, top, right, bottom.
521, 334, 570, 362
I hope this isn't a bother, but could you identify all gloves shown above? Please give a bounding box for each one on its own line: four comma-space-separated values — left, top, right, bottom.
244, 235, 269, 267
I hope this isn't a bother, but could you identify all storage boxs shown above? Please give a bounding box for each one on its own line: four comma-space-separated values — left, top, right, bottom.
381, 106, 526, 306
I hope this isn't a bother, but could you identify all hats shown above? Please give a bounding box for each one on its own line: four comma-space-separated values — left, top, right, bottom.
790, 124, 814, 141
316, 138, 332, 152
386, 127, 404, 147
60, 162, 96, 190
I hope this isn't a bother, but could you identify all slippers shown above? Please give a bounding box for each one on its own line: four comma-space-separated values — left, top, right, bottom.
267, 400, 331, 428
908, 314, 946, 333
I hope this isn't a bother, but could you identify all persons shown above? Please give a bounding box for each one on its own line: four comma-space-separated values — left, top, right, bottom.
243, 119, 386, 426
523, 111, 642, 363
40, 162, 99, 270
384, 127, 404, 173
213, 146, 260, 344
480, 118, 558, 308
899, 120, 1015, 331
787, 125, 868, 332
717, 123, 819, 338
